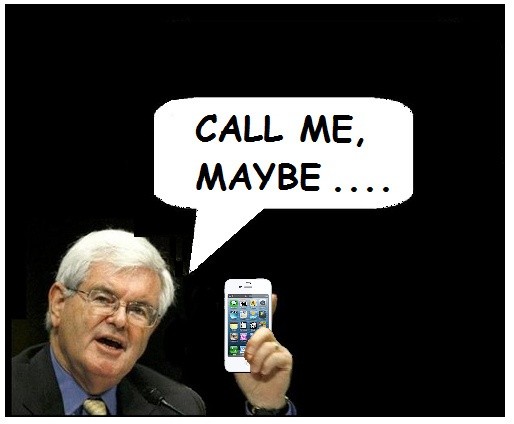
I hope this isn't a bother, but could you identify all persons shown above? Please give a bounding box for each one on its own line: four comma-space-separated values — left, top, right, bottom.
11, 227, 301, 416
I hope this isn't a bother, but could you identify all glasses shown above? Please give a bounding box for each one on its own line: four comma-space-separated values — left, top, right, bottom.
66, 287, 161, 327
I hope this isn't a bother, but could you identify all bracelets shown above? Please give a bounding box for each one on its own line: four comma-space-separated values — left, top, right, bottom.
247, 395, 291, 415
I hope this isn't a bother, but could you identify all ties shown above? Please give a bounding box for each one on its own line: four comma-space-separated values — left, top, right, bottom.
83, 400, 107, 415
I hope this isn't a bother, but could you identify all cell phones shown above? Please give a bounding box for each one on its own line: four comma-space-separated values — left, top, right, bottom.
222, 277, 272, 373
